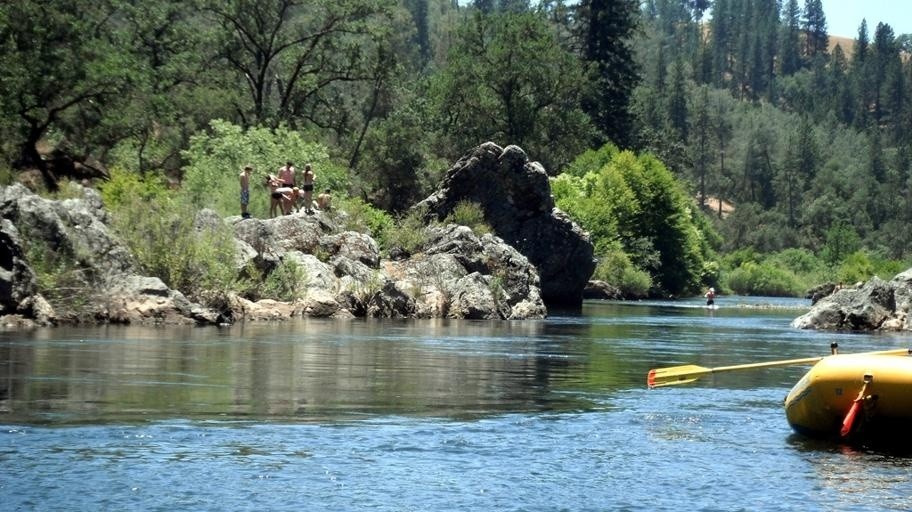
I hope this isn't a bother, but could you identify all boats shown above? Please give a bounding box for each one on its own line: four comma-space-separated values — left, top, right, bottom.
785, 355, 911, 441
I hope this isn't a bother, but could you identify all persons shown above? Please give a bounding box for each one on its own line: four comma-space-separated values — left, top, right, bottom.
278, 160, 296, 214
239, 166, 252, 218
301, 164, 316, 214
704, 287, 715, 306
272, 187, 294, 215
265, 174, 284, 218
312, 188, 333, 210
293, 186, 305, 210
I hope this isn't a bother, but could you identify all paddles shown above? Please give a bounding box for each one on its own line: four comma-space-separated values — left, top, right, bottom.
647, 349, 912, 387
